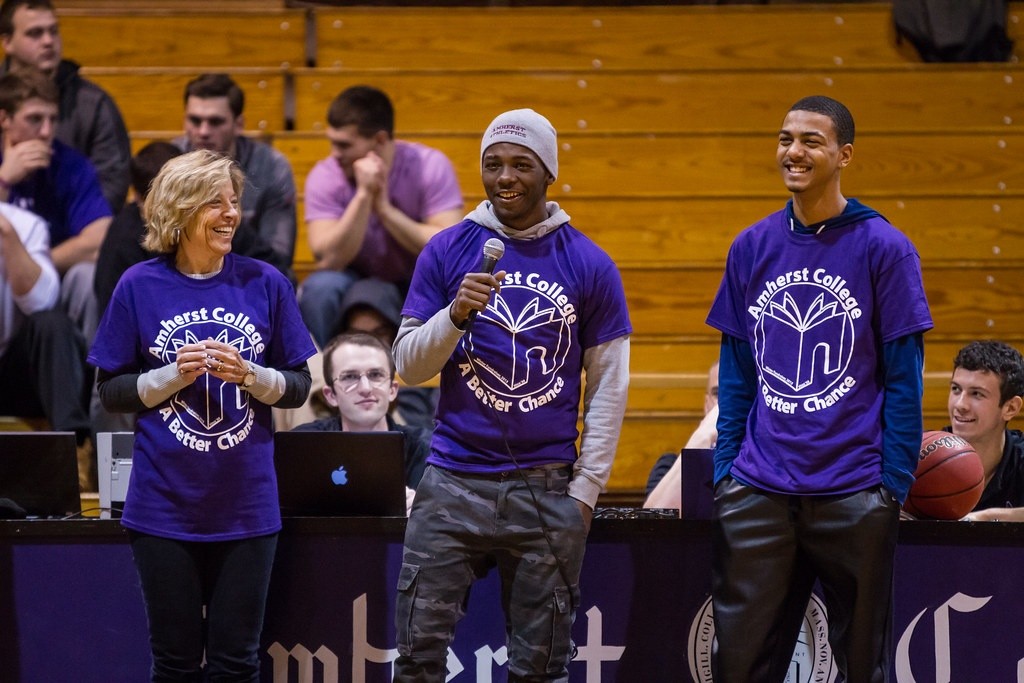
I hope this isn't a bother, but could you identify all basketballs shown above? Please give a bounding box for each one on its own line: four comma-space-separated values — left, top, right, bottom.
900, 430, 987, 522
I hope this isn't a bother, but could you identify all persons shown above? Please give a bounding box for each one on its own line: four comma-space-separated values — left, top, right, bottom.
86, 150, 319, 682
88, 73, 296, 434
389, 107, 630, 683
644, 362, 720, 518
298, 85, 464, 427
939, 340, 1024, 522
0, 0, 132, 491
292, 331, 428, 517
705, 95, 934, 683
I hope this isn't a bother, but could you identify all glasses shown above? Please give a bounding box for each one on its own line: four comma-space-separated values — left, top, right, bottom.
331, 366, 395, 393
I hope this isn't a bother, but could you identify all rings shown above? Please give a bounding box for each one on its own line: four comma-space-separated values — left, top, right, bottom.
217, 362, 222, 372
179, 366, 183, 374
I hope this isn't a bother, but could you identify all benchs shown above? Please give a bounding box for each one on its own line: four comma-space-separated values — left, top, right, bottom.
0, 0, 1024, 499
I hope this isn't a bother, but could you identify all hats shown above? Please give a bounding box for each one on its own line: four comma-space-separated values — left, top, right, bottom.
479, 107, 558, 183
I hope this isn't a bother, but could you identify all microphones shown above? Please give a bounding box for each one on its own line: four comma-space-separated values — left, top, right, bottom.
468, 238, 506, 324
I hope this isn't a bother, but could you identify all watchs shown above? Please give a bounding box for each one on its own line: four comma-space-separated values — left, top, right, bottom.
239, 365, 256, 390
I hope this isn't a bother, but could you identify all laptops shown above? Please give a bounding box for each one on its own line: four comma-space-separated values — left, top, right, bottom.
681, 448, 717, 520
274, 430, 407, 518
0, 431, 81, 519
97, 431, 137, 519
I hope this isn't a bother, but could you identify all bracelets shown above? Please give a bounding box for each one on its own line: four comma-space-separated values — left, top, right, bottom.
0, 179, 12, 188
